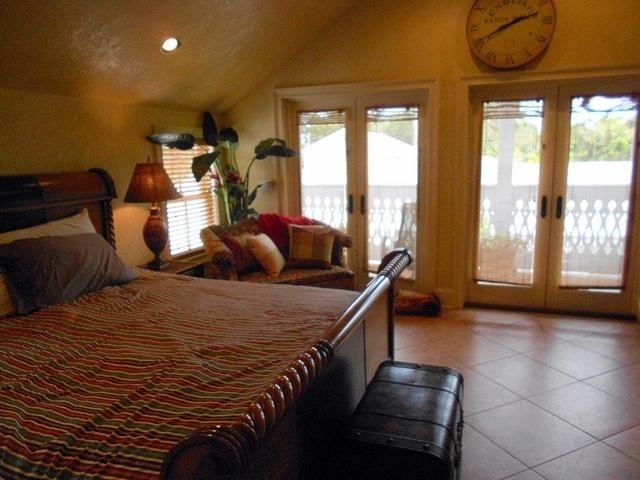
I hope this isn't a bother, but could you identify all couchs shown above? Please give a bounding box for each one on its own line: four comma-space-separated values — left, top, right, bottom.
200, 214, 355, 291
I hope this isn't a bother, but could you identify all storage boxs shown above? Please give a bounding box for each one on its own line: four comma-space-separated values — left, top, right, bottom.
332, 360, 464, 480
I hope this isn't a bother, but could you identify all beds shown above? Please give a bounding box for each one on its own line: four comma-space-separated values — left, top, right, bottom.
1, 167, 414, 479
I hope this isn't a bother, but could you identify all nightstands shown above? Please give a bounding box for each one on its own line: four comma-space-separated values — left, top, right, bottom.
136, 259, 203, 280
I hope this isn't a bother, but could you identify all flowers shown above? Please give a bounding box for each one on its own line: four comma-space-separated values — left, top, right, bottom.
144, 111, 299, 226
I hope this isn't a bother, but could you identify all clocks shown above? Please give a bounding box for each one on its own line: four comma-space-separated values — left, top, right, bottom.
466, 0, 556, 83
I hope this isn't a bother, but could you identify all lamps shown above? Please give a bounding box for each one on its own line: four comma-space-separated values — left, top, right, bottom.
123, 156, 183, 269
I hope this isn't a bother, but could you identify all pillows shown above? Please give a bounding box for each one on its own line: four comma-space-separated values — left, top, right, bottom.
287, 223, 333, 269
258, 213, 312, 247
221, 217, 260, 276
245, 233, 287, 276
0, 208, 97, 325
0, 233, 140, 316
